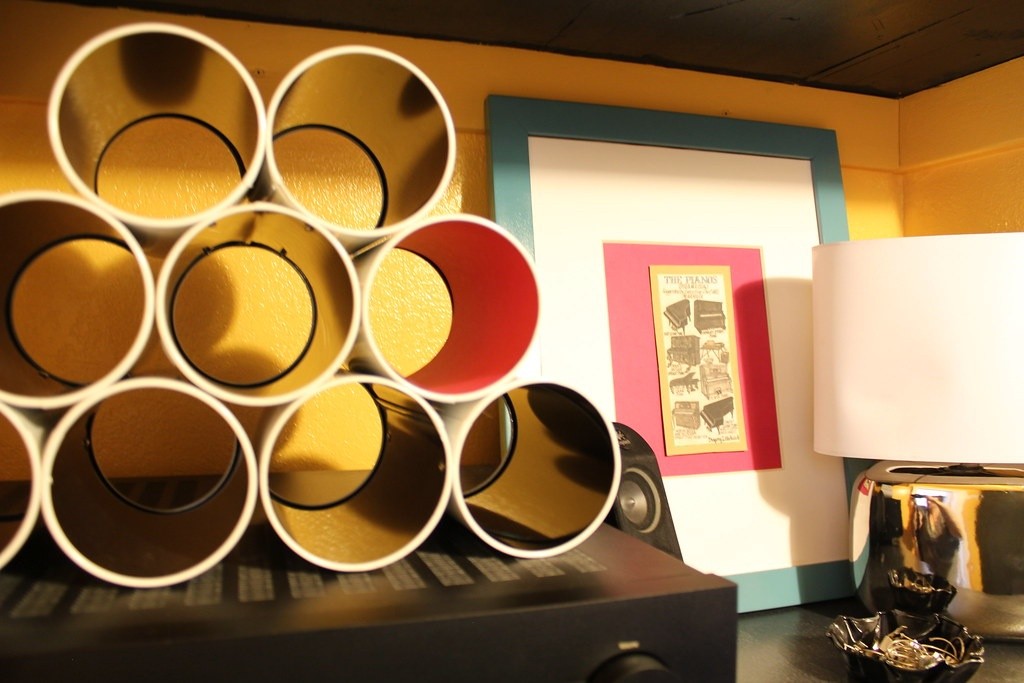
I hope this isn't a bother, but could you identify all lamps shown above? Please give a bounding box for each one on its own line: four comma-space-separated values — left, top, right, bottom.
811, 232, 1024, 642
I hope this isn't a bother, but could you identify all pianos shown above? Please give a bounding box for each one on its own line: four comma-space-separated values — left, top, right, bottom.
664, 297, 736, 435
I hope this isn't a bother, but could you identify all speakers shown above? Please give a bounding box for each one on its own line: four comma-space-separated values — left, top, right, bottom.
610, 420, 684, 563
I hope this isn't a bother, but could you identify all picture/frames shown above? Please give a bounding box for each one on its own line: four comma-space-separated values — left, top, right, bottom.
484, 95, 880, 617
